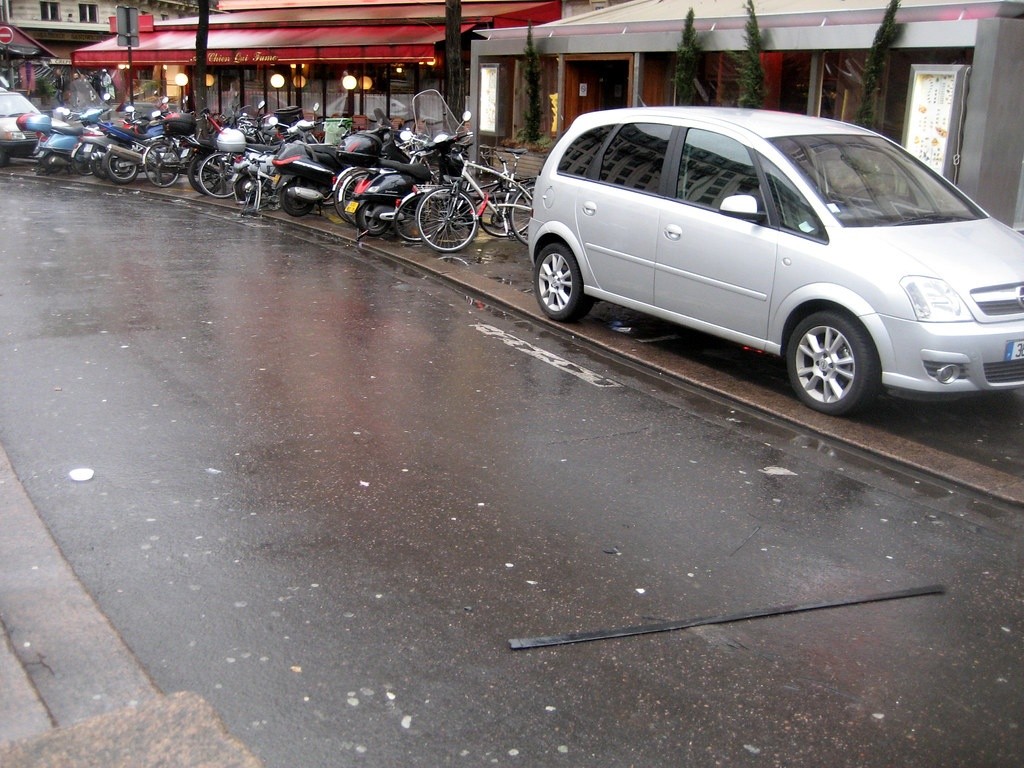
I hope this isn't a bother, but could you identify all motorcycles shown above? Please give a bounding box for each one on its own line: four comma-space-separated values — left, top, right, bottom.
16, 71, 539, 251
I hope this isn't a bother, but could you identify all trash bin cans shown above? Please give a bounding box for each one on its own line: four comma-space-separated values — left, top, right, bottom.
323, 117, 353, 145
274, 105, 303, 133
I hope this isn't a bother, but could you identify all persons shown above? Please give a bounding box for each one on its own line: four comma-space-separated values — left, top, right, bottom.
121, 97, 131, 112
332, 108, 395, 131
335, 65, 394, 113
52, 67, 111, 107
157, 89, 196, 118
67, 13, 76, 23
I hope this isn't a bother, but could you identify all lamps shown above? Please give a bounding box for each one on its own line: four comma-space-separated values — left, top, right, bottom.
343, 75, 357, 91
206, 73, 214, 87
270, 74, 285, 88
293, 75, 306, 87
358, 76, 372, 90
174, 72, 188, 86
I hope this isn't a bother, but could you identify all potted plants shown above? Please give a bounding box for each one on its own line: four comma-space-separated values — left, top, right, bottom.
492, 18, 554, 179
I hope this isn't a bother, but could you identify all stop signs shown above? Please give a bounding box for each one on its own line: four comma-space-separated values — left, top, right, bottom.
0, 27, 14, 45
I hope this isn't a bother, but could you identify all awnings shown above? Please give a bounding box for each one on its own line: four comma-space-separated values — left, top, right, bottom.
0, 25, 60, 58
67, 22, 476, 67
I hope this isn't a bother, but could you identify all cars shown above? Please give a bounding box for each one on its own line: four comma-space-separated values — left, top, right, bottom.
0, 87, 46, 164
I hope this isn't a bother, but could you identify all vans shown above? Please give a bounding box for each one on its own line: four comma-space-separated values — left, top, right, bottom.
529, 107, 1024, 419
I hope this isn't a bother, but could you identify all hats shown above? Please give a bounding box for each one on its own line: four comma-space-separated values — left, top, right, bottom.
102, 69, 107, 72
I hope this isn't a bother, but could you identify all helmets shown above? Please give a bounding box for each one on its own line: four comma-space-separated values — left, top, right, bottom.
53, 107, 71, 122
